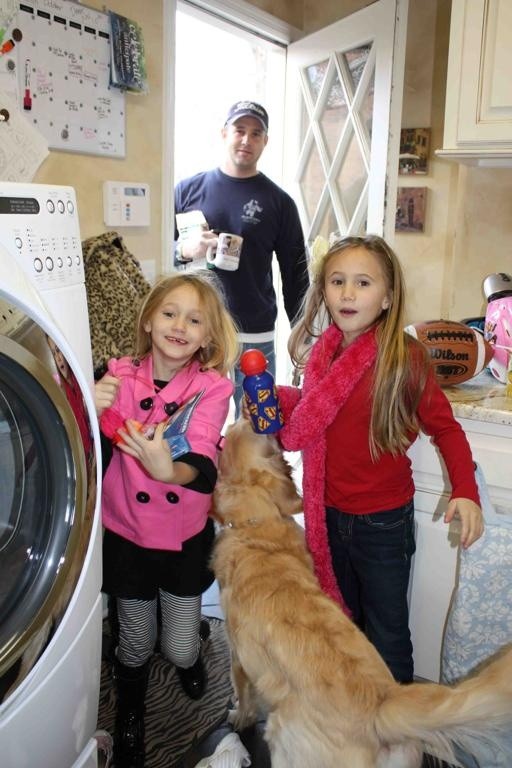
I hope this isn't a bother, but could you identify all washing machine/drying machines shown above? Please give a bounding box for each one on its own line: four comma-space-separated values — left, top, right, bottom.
0, 178, 110, 765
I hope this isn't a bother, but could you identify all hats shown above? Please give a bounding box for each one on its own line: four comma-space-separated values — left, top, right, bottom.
225, 101, 269, 132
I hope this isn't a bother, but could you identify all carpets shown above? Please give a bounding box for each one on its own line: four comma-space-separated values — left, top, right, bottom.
86, 610, 461, 768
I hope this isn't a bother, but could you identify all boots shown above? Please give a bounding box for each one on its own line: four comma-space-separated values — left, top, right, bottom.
173, 649, 206, 701
112, 659, 149, 768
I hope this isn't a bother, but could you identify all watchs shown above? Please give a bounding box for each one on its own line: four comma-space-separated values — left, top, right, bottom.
175, 240, 192, 263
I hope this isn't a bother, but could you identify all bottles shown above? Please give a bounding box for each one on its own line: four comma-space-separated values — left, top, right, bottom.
238, 347, 287, 436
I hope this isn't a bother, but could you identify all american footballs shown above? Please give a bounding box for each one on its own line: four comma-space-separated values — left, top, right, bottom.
404, 320, 496, 388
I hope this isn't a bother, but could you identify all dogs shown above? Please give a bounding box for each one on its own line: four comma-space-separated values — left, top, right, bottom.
207, 415, 512, 768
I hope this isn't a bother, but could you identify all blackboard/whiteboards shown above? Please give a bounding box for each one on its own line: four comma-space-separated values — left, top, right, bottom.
0, 0, 127, 161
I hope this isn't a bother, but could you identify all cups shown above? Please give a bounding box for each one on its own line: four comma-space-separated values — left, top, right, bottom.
205, 232, 244, 272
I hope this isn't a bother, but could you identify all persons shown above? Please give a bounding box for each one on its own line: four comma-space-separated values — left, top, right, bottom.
95, 270, 239, 768
243, 235, 486, 685
174, 99, 314, 419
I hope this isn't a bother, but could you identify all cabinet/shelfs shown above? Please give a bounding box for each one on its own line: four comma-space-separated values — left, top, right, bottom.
406, 418, 512, 680
435, 0, 512, 166
